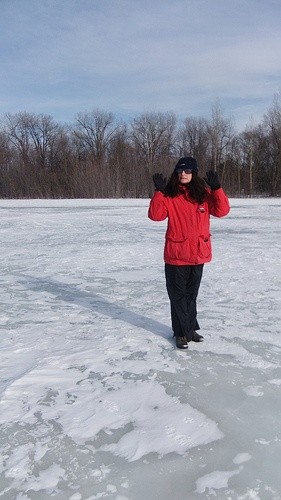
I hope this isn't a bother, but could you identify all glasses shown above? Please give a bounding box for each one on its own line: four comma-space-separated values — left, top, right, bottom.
176, 167, 194, 173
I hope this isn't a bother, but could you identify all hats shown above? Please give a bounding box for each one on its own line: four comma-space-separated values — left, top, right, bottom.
176, 158, 197, 169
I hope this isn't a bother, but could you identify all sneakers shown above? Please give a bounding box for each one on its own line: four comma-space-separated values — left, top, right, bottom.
176, 336, 188, 349
192, 331, 204, 342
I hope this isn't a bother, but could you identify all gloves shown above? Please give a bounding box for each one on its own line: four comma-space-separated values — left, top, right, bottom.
203, 170, 220, 189
153, 173, 167, 191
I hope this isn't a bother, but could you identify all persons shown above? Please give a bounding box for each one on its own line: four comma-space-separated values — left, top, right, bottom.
148, 157, 230, 349
